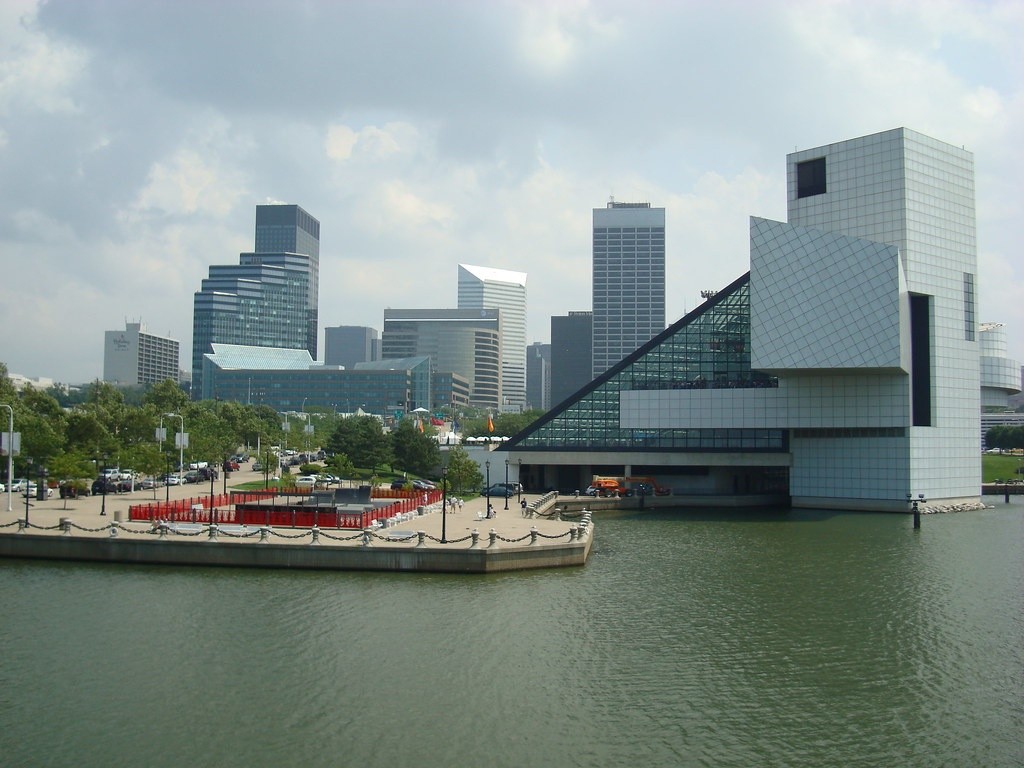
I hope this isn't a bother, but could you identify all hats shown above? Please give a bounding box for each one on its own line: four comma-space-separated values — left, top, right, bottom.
460, 497, 462, 499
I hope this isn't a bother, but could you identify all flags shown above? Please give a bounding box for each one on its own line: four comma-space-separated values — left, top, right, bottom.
453, 416, 461, 433
488, 414, 496, 433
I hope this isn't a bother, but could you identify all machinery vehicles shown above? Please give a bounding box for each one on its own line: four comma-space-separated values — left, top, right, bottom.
592, 475, 671, 496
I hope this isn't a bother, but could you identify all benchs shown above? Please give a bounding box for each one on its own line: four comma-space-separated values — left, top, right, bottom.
165, 523, 203, 533
396, 512, 406, 521
372, 519, 383, 528
218, 524, 271, 537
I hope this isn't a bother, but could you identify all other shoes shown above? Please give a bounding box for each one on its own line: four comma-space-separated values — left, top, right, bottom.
450, 512, 452, 514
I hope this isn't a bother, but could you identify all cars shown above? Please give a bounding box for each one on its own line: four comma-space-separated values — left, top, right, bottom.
296, 474, 341, 487
1, 453, 254, 500
252, 446, 325, 470
585, 485, 596, 495
391, 480, 424, 491
412, 480, 436, 490
483, 482, 523, 498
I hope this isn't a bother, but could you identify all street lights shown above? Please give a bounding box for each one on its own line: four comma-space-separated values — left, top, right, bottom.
160, 413, 183, 485
26, 456, 33, 528
165, 449, 171, 503
207, 464, 215, 536
484, 459, 492, 519
504, 459, 508, 510
518, 458, 522, 503
100, 451, 109, 515
247, 378, 402, 451
440, 467, 447, 543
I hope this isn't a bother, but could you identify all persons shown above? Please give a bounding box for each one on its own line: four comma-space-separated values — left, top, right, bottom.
459, 497, 464, 511
450, 495, 457, 513
489, 504, 496, 518
440, 499, 448, 515
521, 498, 527, 516
